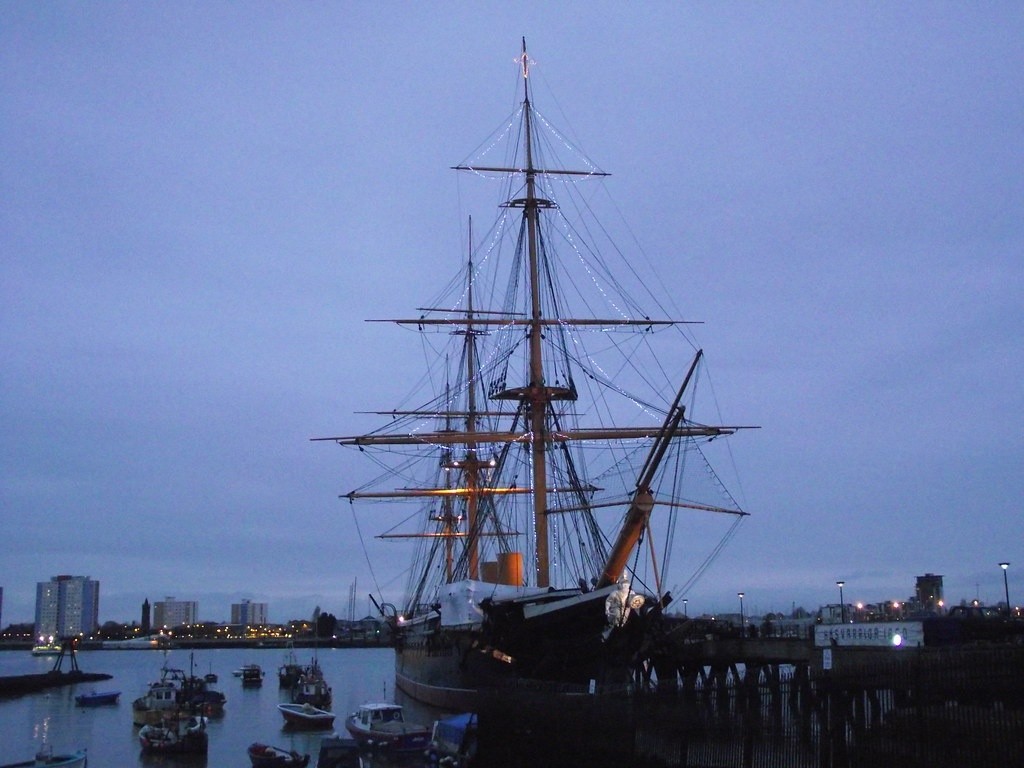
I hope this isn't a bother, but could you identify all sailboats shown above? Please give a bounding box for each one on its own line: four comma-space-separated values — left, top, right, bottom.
308, 38, 762, 714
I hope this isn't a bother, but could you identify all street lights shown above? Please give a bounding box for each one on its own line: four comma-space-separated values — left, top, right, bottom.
836, 580, 845, 625
737, 592, 744, 641
998, 562, 1009, 618
682, 598, 688, 621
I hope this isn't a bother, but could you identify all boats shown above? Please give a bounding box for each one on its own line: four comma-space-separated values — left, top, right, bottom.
129, 651, 226, 757
75, 690, 121, 707
278, 703, 336, 730
247, 743, 311, 768
277, 642, 307, 685
345, 703, 433, 752
231, 667, 266, 678
316, 734, 363, 768
33, 646, 77, 656
292, 658, 333, 708
0, 742, 88, 768
239, 665, 263, 688
